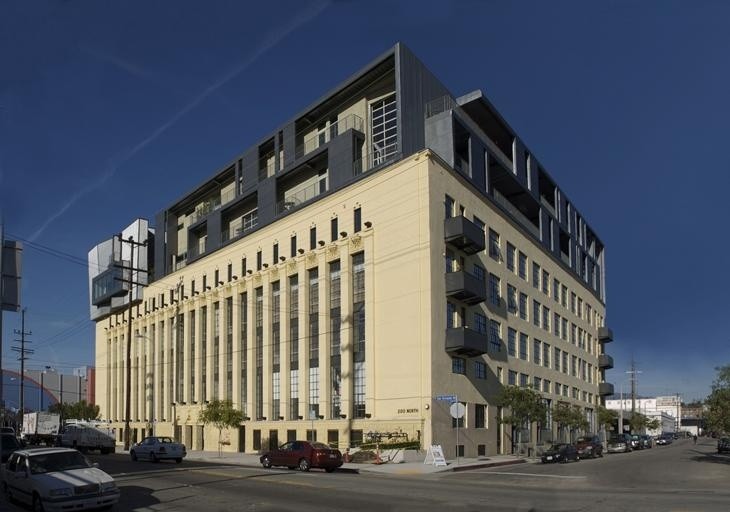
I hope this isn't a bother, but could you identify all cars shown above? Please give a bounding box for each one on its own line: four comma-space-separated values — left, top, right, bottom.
0, 436, 24, 482
0, 448, 122, 511
540, 442, 581, 463
715, 435, 730, 457
257, 441, 344, 475
656, 436, 672, 445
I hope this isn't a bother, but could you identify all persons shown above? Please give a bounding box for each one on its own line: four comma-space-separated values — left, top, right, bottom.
693, 434, 697, 444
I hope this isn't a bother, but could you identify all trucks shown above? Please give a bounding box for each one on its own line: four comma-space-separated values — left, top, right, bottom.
17, 412, 63, 446
60, 419, 116, 455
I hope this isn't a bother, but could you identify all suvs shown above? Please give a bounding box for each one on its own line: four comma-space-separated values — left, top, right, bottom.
606, 433, 653, 454
1, 426, 16, 435
571, 435, 604, 458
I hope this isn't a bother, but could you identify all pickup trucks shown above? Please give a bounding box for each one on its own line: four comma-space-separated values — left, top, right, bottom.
129, 436, 187, 466
